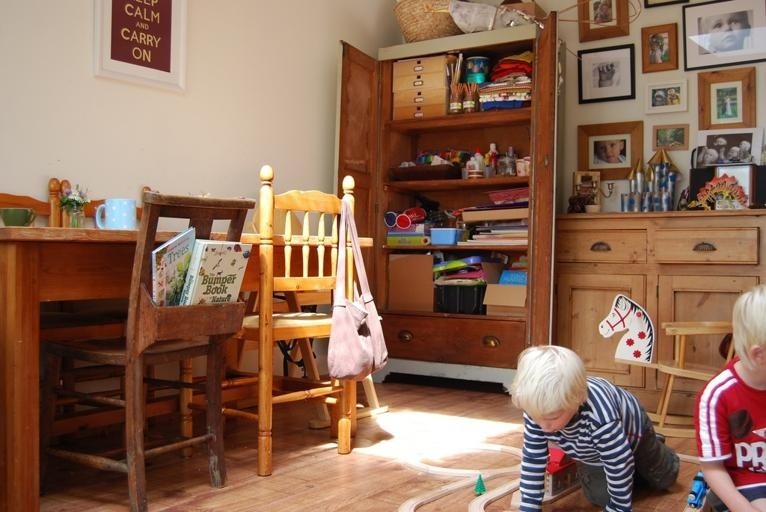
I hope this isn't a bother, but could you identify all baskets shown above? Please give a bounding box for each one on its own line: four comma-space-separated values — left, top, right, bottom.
393, 0, 465, 43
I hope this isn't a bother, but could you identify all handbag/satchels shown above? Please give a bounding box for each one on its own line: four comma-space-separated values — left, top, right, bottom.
327, 293, 389, 381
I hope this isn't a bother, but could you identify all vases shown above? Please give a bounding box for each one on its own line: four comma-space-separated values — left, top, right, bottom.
65, 206, 84, 229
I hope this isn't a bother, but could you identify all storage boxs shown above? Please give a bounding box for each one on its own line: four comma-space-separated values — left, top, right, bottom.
387, 251, 435, 315
482, 282, 529, 320
391, 55, 451, 120
428, 225, 467, 246
433, 284, 487, 317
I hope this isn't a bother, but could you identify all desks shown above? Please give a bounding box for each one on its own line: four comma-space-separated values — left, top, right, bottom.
0, 225, 373, 511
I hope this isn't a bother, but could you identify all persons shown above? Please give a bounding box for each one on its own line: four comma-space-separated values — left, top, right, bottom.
667, 89, 680, 105
655, 91, 665, 106
694, 285, 766, 512
701, 11, 751, 54
722, 97, 726, 115
595, 139, 626, 164
725, 95, 732, 115
697, 137, 750, 168
506, 347, 677, 512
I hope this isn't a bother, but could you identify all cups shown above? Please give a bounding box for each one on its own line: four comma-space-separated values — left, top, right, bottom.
468, 169, 484, 180
515, 158, 531, 177
584, 204, 601, 213
95, 198, 138, 230
480, 261, 504, 284
485, 167, 496, 178
450, 93, 479, 114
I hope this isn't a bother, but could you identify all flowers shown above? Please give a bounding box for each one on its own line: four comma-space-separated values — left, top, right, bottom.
57, 184, 91, 225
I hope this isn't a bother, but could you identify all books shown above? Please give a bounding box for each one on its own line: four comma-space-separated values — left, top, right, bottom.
152, 227, 195, 307
178, 239, 252, 306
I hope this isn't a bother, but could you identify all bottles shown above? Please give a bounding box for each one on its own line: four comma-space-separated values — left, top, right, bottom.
499, 146, 519, 176
467, 142, 500, 170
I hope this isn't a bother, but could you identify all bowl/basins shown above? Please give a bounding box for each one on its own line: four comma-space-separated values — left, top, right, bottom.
0, 207, 37, 226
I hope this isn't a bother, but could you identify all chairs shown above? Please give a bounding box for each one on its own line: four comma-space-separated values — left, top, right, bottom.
62, 179, 152, 228
0, 175, 61, 226
645, 320, 736, 437
220, 162, 360, 476
39, 185, 258, 512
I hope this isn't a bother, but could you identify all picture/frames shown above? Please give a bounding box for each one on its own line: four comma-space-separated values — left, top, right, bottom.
642, 22, 678, 75
712, 163, 758, 211
681, 4, 766, 73
576, 40, 637, 107
643, 0, 690, 10
697, 66, 761, 131
576, 119, 646, 182
642, 78, 689, 115
652, 123, 692, 152
576, 0, 630, 44
89, 0, 189, 98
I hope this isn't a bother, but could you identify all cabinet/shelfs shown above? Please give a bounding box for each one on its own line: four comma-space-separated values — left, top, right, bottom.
332, 8, 562, 394
554, 206, 766, 416
